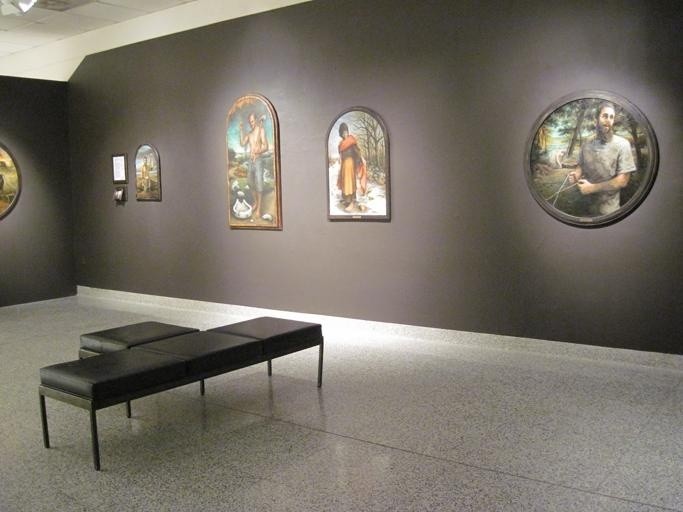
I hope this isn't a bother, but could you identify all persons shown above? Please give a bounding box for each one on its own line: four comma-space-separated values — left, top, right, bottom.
237, 113, 268, 218
564, 101, 639, 217
335, 122, 365, 214
139, 156, 153, 193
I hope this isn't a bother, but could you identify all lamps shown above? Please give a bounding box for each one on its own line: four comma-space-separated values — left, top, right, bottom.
0, 0, 38, 18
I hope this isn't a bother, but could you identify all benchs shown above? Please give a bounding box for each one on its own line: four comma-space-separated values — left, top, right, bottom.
37, 315, 324, 471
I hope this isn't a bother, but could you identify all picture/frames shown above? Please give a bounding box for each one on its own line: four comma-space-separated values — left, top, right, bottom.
521, 87, 660, 230
111, 151, 129, 185
133, 143, 162, 201
224, 92, 283, 231
0, 142, 22, 220
323, 105, 391, 223
